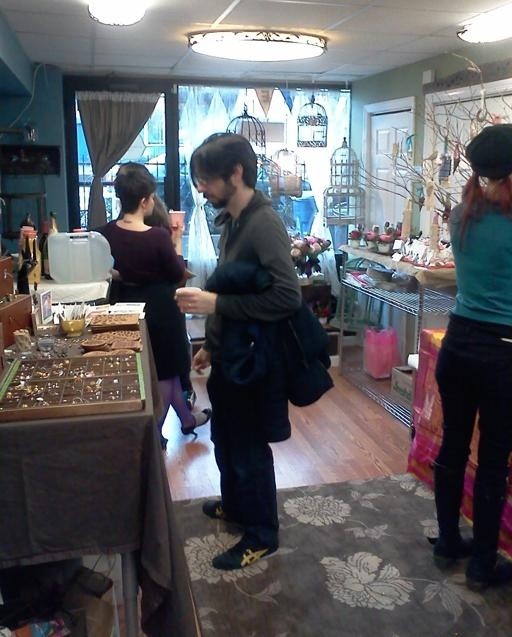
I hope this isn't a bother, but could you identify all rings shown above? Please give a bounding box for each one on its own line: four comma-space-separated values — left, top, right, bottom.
188, 303, 191, 307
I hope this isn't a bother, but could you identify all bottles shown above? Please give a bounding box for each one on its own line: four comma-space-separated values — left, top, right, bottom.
18, 211, 36, 243
38, 209, 60, 280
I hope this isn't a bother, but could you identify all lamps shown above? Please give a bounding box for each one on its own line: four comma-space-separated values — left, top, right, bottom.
184, 29, 326, 62
457, 2, 511, 43
86, 2, 148, 27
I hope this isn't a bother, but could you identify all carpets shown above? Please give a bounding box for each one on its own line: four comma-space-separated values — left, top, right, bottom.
149, 469, 510, 635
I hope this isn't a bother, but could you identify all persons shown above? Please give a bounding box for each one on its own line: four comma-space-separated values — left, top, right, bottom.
434, 125, 512, 590
114, 162, 196, 410
173, 133, 302, 569
95, 169, 211, 450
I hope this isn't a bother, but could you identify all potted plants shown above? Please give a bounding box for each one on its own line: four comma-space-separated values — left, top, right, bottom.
345, 222, 402, 255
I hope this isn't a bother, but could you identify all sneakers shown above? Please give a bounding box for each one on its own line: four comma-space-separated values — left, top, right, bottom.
212, 535, 280, 571
203, 499, 226, 522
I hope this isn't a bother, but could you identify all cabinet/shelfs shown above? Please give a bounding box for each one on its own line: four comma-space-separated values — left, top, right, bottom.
0, 245, 195, 636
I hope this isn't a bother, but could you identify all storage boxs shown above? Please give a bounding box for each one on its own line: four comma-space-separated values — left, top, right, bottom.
390, 364, 415, 403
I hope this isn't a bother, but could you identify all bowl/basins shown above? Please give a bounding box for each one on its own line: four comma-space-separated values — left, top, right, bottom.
60, 318, 85, 338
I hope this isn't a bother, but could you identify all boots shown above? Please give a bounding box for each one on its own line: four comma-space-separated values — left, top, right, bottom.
466, 472, 512, 592
431, 461, 476, 569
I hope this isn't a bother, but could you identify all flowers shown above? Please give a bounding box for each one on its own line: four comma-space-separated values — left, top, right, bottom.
288, 231, 333, 274
315, 299, 331, 317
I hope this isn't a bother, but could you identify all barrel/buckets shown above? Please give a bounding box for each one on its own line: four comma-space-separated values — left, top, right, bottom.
364, 326, 401, 379
46, 231, 114, 283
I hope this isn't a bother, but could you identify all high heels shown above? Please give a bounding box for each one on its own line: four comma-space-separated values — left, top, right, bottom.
181, 408, 211, 436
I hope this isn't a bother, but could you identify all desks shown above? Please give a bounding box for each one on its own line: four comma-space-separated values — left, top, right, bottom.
404, 324, 512, 561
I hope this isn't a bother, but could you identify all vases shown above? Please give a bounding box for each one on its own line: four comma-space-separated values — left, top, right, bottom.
318, 316, 327, 326
297, 256, 306, 277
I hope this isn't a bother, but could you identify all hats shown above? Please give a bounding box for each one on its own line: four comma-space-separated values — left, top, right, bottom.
465, 124, 512, 177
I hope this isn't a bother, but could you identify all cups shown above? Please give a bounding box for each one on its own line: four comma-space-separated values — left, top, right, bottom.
175, 286, 202, 313
168, 208, 186, 228
